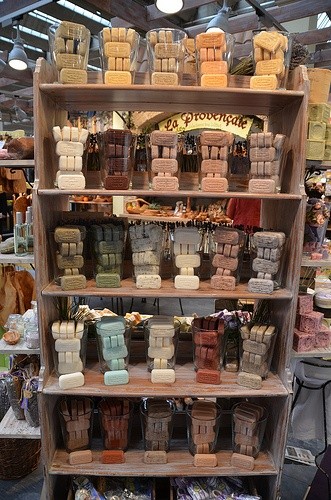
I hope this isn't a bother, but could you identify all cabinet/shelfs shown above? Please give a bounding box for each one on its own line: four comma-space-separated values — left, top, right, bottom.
33, 57, 310, 500
1, 160, 40, 439
291, 160, 331, 357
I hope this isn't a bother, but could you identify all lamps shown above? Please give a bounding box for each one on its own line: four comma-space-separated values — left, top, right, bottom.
8, 21, 28, 71
154, 0, 185, 15
204, 0, 237, 33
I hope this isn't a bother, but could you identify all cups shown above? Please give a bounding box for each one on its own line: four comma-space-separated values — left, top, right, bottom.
146, 133, 186, 191
187, 399, 222, 455
95, 129, 139, 190
195, 131, 235, 193
250, 30, 293, 91
94, 321, 131, 373
97, 26, 140, 85
139, 398, 176, 453
143, 318, 181, 371
249, 233, 288, 290
145, 28, 189, 86
57, 396, 94, 451
238, 322, 279, 380
230, 402, 269, 460
48, 23, 90, 84
47, 227, 206, 285
48, 320, 87, 378
247, 132, 290, 193
206, 230, 247, 286
49, 125, 91, 190
96, 397, 134, 452
3, 313, 41, 350
195, 32, 234, 88
305, 197, 331, 320
191, 318, 229, 372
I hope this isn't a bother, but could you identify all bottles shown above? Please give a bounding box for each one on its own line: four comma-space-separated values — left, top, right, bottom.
13, 207, 34, 258
